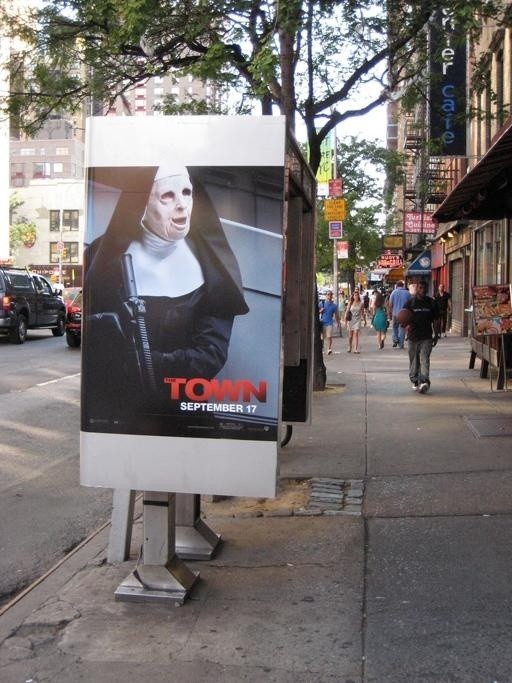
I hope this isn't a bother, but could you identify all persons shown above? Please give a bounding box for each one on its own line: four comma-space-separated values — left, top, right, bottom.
316, 280, 416, 355
399, 281, 439, 393
433, 283, 453, 338
82, 166, 250, 425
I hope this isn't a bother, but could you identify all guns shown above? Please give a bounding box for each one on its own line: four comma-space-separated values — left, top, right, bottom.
89, 251, 161, 409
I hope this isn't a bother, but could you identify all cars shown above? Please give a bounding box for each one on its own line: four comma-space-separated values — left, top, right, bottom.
65, 289, 82, 348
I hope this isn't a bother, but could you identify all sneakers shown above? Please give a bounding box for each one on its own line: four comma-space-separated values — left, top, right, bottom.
420, 383, 429, 393
327, 350, 332, 355
348, 347, 352, 352
381, 340, 384, 349
392, 339, 399, 347
355, 351, 360, 354
412, 382, 419, 391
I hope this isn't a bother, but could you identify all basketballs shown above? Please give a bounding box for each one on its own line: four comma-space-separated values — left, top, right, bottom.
397, 309, 412, 325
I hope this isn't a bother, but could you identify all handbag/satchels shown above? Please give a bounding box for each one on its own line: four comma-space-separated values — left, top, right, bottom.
347, 312, 352, 321
319, 318, 324, 332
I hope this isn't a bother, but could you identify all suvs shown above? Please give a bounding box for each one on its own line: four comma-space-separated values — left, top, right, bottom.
0, 266, 66, 343
